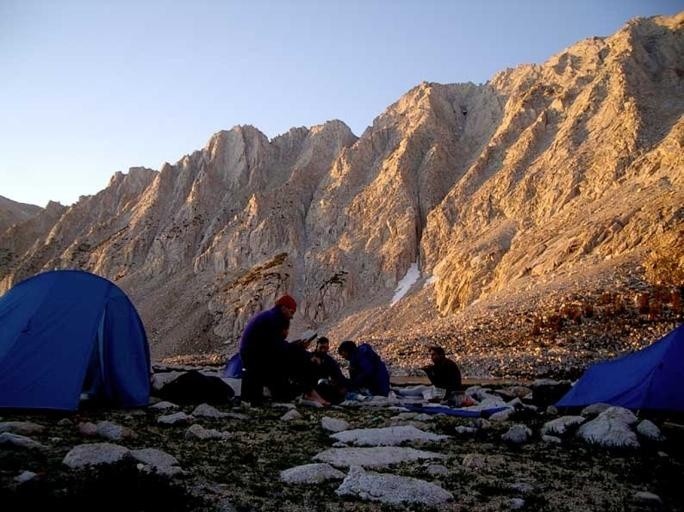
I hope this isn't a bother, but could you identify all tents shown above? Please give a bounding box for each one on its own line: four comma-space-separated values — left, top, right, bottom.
557, 322, 684, 407
0, 270, 151, 411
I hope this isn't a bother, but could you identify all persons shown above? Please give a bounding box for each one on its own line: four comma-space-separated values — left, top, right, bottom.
239, 294, 390, 405
418, 347, 462, 404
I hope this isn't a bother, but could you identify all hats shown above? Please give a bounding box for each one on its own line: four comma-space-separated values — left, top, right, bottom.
273, 295, 296, 310
